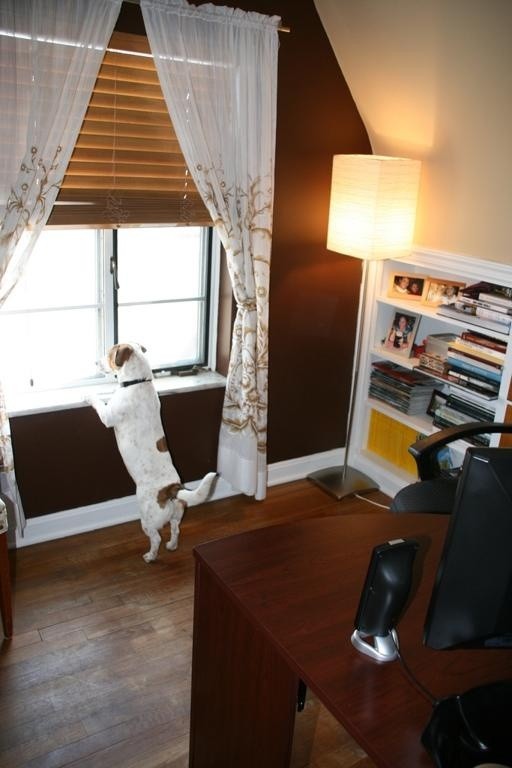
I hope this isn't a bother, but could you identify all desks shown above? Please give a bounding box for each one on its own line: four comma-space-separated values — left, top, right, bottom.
191, 501, 512, 768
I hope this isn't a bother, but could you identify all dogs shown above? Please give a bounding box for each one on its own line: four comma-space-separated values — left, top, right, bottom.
79, 341, 218, 566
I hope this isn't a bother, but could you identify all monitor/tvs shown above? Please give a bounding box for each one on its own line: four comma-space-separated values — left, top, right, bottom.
422, 446, 512, 651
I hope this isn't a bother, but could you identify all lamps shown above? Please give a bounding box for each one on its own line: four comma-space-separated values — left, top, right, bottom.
306, 154, 422, 500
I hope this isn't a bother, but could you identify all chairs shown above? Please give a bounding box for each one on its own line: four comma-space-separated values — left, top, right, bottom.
389, 422, 512, 512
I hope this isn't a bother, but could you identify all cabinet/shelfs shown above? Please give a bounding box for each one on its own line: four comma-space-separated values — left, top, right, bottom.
341, 248, 512, 504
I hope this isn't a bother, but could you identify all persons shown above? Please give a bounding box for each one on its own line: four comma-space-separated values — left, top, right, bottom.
394, 277, 411, 293
406, 278, 420, 295
384, 311, 414, 349
438, 283, 458, 306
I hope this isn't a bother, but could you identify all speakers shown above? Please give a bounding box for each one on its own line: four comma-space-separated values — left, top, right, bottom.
350, 538, 420, 664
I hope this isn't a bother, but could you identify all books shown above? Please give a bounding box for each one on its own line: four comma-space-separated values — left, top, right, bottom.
435, 303, 510, 334
363, 329, 509, 483
457, 293, 511, 314
453, 300, 511, 326
459, 280, 511, 308
